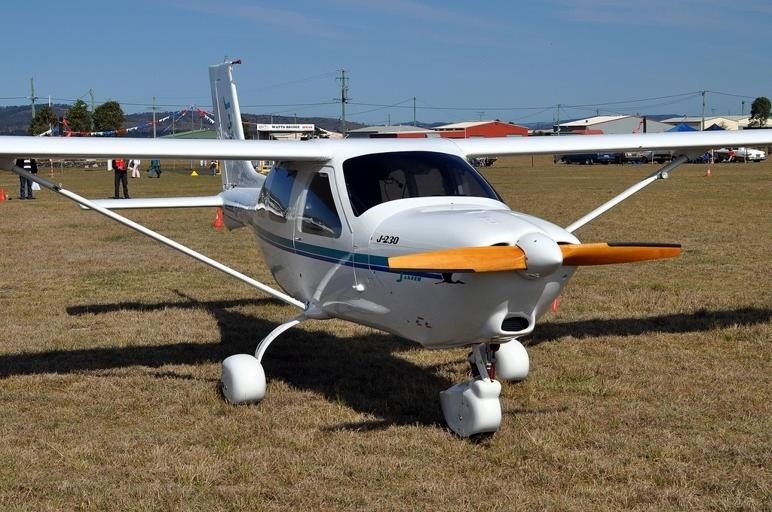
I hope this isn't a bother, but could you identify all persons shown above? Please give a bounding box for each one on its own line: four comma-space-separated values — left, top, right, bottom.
16, 158, 39, 200
112, 158, 131, 200
129, 159, 141, 178
149, 160, 161, 178
210, 160, 218, 177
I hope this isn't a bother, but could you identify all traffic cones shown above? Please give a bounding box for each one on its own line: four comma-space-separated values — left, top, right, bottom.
0, 188, 6, 200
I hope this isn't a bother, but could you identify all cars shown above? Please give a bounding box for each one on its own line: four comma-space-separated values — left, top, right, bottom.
561, 147, 766, 165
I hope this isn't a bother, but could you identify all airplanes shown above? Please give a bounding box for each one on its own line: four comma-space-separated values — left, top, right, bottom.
0, 59, 772, 440
